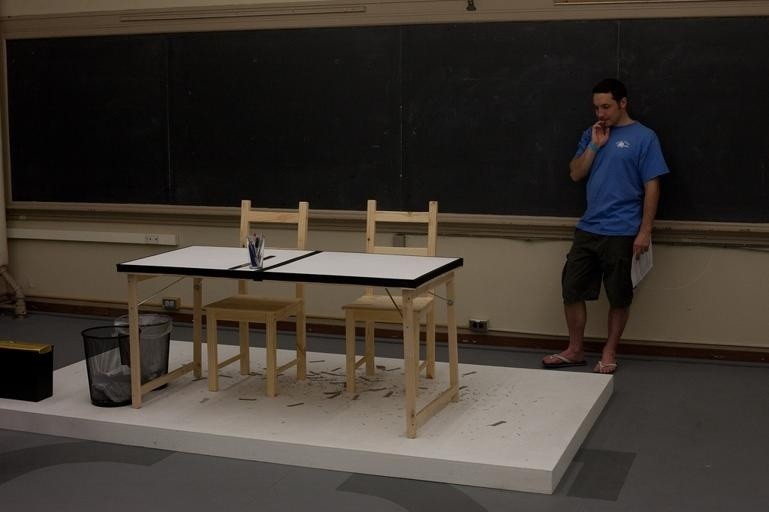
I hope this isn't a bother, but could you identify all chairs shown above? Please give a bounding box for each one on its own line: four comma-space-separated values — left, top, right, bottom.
340, 200, 438, 391
203, 200, 309, 398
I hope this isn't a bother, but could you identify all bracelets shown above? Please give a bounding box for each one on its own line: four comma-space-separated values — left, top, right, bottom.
587, 142, 599, 152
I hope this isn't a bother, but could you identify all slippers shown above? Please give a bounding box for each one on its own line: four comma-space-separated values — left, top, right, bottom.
593, 360, 617, 374
542, 353, 587, 368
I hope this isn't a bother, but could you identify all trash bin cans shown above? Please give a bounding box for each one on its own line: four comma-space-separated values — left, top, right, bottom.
114, 314, 171, 392
81, 325, 142, 407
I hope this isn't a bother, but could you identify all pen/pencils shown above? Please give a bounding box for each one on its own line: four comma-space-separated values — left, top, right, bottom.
247, 231, 267, 269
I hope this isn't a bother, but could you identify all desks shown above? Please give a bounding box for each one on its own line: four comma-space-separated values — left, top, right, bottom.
117, 245, 465, 438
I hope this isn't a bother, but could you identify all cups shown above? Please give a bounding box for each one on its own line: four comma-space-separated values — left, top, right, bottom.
247, 235, 264, 269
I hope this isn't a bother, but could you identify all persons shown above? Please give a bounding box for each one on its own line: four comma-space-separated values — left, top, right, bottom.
540, 76, 671, 375
